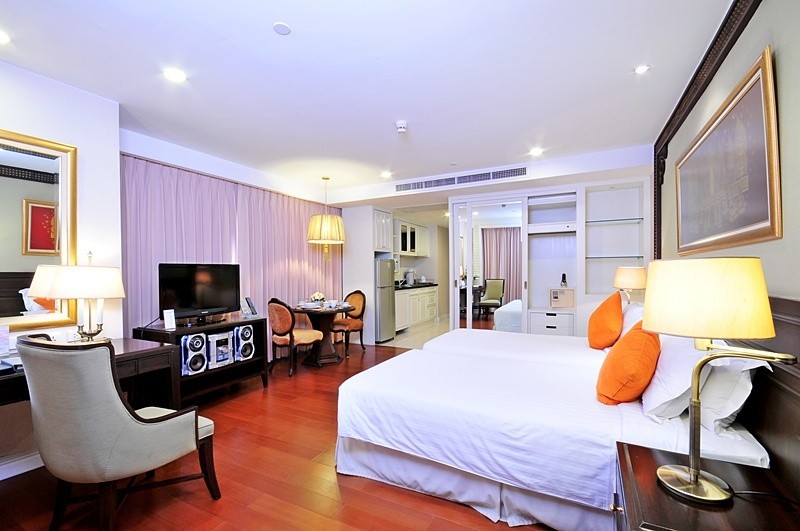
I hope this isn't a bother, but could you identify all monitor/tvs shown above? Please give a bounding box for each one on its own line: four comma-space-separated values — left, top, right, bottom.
158, 263, 240, 320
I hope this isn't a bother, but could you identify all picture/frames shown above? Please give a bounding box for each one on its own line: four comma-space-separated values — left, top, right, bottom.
21, 199, 62, 258
673, 46, 784, 257
246, 297, 259, 315
238, 298, 253, 319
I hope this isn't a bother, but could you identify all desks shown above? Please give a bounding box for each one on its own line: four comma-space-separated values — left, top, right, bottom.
1, 336, 182, 416
473, 285, 485, 320
291, 302, 355, 369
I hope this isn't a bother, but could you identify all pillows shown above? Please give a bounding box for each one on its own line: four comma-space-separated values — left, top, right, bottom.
18, 288, 61, 314
586, 292, 773, 434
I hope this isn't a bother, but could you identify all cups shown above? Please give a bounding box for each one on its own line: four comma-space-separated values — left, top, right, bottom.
322, 302, 330, 308
331, 302, 336, 308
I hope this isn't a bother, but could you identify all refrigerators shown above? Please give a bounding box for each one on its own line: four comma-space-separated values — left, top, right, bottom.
376, 257, 396, 344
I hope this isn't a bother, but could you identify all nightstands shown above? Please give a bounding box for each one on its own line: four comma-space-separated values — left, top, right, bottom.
607, 441, 800, 531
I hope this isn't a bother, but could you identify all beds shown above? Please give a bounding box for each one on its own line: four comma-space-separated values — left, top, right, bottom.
493, 299, 524, 333
335, 292, 799, 531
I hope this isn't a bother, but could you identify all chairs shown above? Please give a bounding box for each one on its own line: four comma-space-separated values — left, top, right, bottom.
329, 290, 367, 359
266, 298, 325, 377
16, 331, 222, 530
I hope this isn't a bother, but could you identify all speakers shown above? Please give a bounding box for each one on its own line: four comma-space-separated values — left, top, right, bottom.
180, 325, 255, 376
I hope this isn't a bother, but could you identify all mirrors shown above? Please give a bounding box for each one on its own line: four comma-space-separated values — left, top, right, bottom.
478, 279, 505, 321
466, 200, 525, 334
0, 128, 80, 332
454, 202, 467, 328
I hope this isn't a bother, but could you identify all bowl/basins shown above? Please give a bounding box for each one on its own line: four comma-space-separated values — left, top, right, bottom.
331, 300, 338, 305
305, 302, 316, 308
342, 301, 349, 307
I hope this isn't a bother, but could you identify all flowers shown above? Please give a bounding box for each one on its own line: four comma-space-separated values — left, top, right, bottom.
311, 292, 326, 303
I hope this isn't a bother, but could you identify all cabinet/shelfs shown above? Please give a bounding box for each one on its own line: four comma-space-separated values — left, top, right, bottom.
395, 220, 417, 257
131, 315, 269, 408
409, 288, 426, 326
415, 225, 429, 258
583, 179, 645, 296
342, 207, 395, 253
426, 287, 438, 320
395, 290, 409, 333
528, 308, 576, 336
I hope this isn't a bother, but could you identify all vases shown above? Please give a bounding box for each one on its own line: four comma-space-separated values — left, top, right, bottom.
313, 301, 323, 306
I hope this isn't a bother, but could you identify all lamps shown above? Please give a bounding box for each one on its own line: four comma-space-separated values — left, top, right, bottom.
59, 264, 127, 346
30, 265, 63, 301
643, 254, 798, 510
613, 266, 648, 306
306, 176, 345, 264
